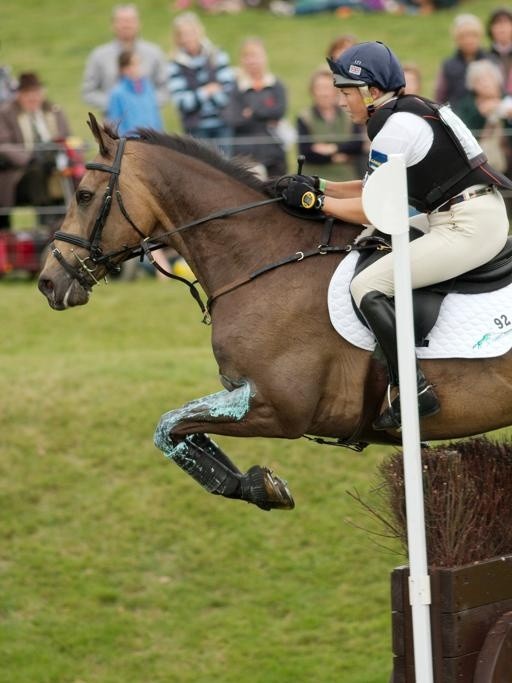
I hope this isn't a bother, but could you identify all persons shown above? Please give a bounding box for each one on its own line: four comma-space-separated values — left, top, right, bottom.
397, 8, 512, 176
269, 40, 509, 432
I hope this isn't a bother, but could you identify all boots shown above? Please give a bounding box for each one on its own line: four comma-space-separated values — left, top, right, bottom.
359, 290, 442, 432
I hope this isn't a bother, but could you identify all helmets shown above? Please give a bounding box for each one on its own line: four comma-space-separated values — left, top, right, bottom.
326, 39, 406, 93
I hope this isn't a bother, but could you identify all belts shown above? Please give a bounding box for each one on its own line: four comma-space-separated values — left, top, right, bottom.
437, 185, 495, 212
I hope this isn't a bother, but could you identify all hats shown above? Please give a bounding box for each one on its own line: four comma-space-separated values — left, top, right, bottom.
8, 73, 49, 93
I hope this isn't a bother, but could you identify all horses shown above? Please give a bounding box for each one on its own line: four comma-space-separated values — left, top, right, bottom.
36, 110, 511, 512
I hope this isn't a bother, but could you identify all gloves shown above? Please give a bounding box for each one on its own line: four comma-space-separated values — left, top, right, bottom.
272, 174, 326, 216
